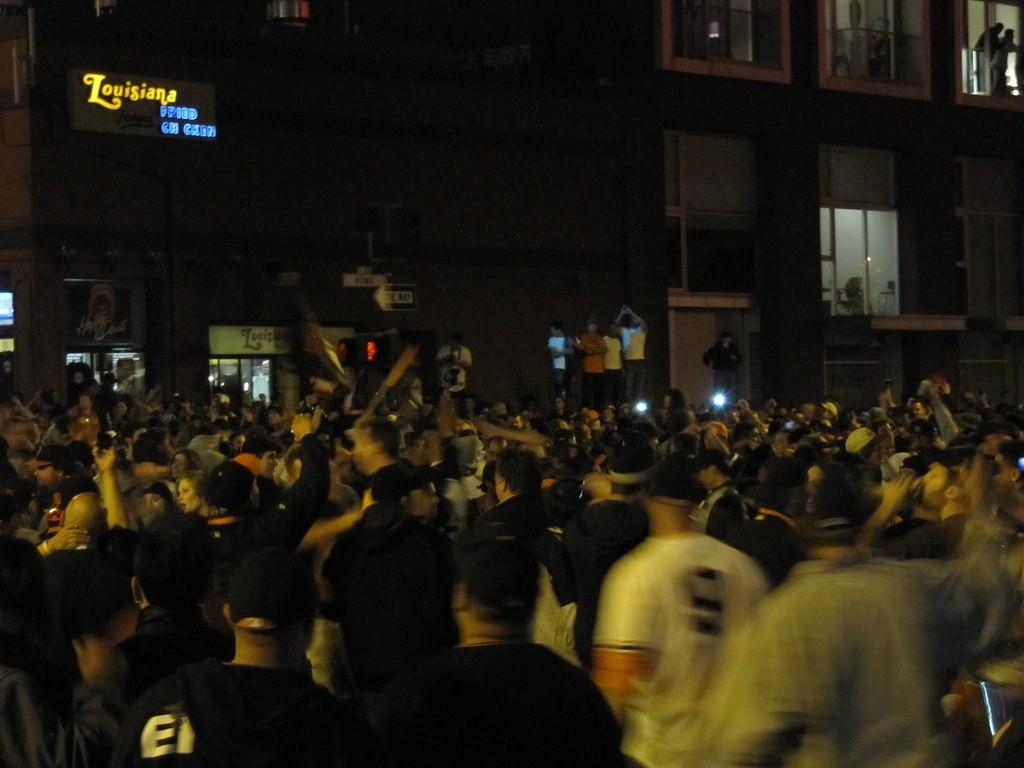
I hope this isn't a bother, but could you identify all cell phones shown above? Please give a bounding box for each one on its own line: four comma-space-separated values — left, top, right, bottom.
96, 433, 112, 450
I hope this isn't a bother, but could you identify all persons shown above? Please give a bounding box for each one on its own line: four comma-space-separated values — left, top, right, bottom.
1, 303, 1024, 768
976, 23, 1019, 93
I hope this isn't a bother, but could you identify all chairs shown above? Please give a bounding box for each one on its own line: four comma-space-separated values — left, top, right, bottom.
879, 281, 896, 312
838, 276, 863, 312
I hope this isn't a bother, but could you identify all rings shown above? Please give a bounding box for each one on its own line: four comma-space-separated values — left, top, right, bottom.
299, 414, 303, 416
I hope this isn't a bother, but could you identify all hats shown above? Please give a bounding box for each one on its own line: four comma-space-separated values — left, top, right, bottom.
908, 418, 935, 435
694, 449, 724, 475
845, 427, 889, 459
226, 544, 316, 633
52, 477, 98, 511
142, 481, 173, 506
723, 403, 743, 411
811, 507, 850, 531
26, 446, 66, 467
447, 537, 538, 608
600, 433, 656, 483
821, 402, 838, 416
133, 438, 167, 466
208, 461, 256, 511
372, 462, 429, 501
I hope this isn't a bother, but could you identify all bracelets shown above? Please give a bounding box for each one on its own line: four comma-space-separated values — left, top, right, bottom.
43, 539, 53, 555
97, 468, 115, 476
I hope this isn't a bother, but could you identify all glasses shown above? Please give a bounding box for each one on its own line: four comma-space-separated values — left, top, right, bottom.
36, 463, 56, 471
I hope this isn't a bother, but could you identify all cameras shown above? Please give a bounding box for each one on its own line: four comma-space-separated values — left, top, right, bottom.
785, 421, 795, 430
